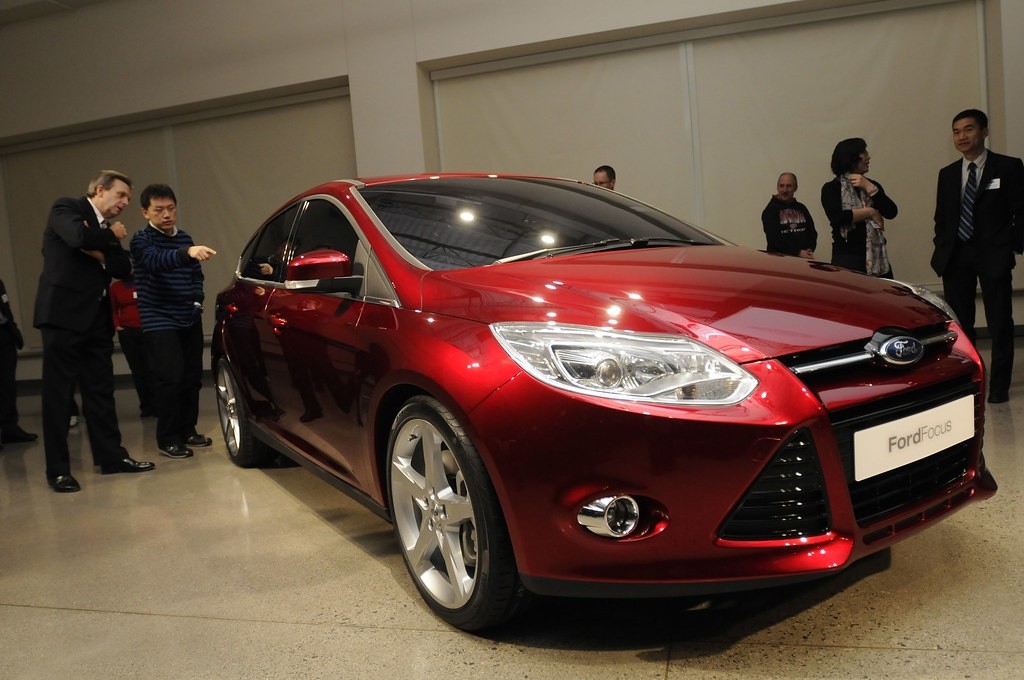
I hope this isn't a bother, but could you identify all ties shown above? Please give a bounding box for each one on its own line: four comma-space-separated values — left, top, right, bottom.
957, 162, 977, 241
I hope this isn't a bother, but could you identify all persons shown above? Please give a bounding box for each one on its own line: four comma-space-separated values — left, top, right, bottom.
0, 279, 39, 444
110, 275, 158, 417
761, 172, 818, 259
32, 169, 155, 493
130, 184, 218, 459
821, 138, 898, 279
242, 235, 300, 282
593, 165, 616, 190
930, 109, 1024, 403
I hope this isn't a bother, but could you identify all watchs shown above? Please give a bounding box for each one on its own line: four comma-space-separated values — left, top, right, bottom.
868, 185, 877, 195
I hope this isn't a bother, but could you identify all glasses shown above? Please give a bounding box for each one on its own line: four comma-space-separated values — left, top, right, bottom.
592, 180, 610, 185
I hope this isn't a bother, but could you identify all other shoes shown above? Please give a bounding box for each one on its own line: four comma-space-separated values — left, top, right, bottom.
183, 434, 212, 447
158, 442, 193, 459
69, 415, 78, 426
4, 430, 38, 443
987, 394, 1009, 404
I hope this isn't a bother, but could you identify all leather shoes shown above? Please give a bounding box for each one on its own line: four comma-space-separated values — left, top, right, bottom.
47, 472, 80, 493
101, 456, 155, 474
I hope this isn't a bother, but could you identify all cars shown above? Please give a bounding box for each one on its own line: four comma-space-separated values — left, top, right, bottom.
211, 174, 998, 635
